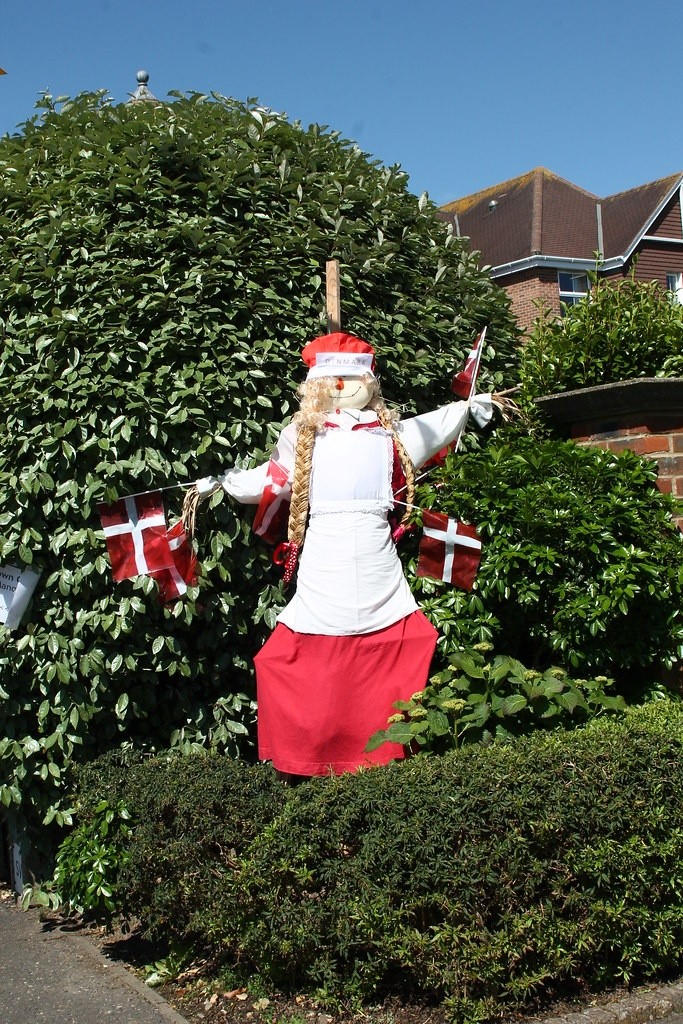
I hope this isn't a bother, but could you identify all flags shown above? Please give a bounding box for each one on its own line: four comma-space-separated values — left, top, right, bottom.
417, 508, 483, 594
143, 520, 198, 605
252, 457, 293, 546
451, 328, 485, 399
96, 489, 176, 582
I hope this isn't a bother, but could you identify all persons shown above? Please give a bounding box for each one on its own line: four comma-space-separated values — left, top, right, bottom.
190, 332, 495, 776
